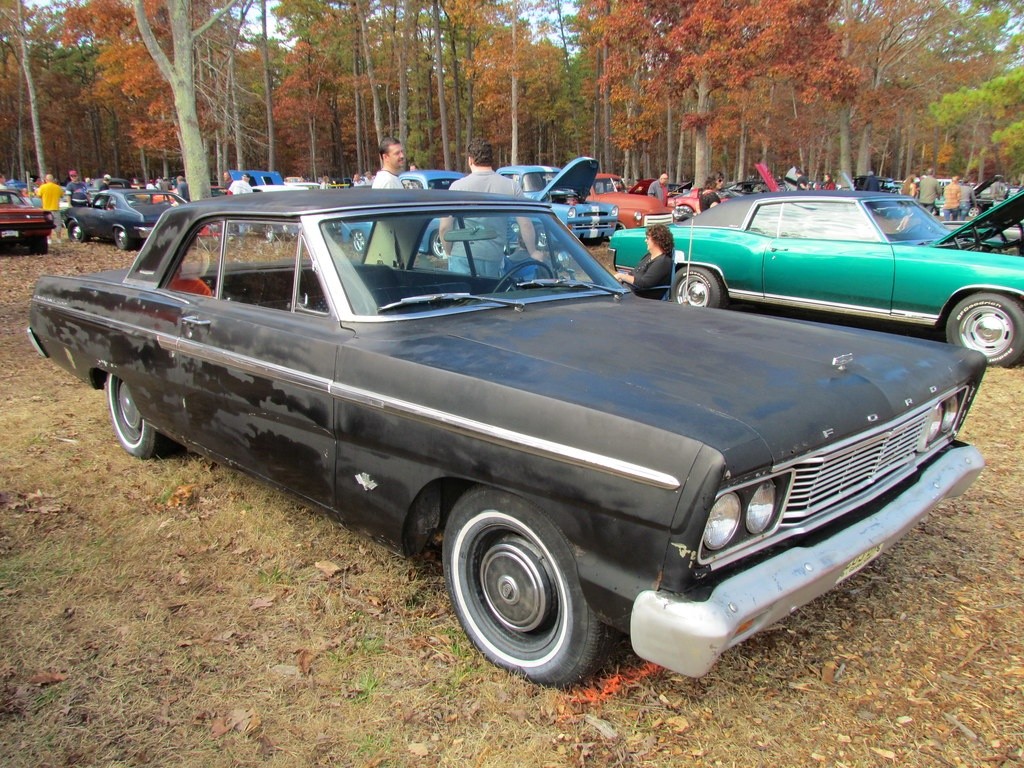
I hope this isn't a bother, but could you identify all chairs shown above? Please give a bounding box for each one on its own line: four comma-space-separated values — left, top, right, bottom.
631, 249, 676, 303
194, 222, 222, 257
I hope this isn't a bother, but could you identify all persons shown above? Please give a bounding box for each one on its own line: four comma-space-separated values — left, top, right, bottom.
614, 224, 675, 300
794, 170, 879, 192
699, 171, 725, 213
409, 163, 419, 172
320, 171, 375, 190
439, 139, 543, 277
224, 171, 252, 235
647, 173, 672, 207
901, 167, 977, 221
0, 170, 112, 244
372, 136, 405, 189
146, 176, 191, 203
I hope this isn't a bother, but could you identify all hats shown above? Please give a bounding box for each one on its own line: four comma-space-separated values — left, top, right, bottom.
104, 174, 111, 180
69, 170, 77, 175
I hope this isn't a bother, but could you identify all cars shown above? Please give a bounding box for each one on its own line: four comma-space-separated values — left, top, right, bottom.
607, 191, 1024, 369
495, 157, 619, 254
340, 170, 520, 258
585, 173, 679, 243
25, 188, 988, 689
62, 188, 188, 251
0, 190, 57, 255
628, 163, 1024, 218
4, 170, 352, 237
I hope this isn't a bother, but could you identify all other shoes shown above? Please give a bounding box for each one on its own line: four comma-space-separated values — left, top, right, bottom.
56, 238, 61, 244
47, 239, 52, 244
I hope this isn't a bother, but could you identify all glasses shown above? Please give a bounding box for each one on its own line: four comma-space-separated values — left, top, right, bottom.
718, 179, 722, 182
